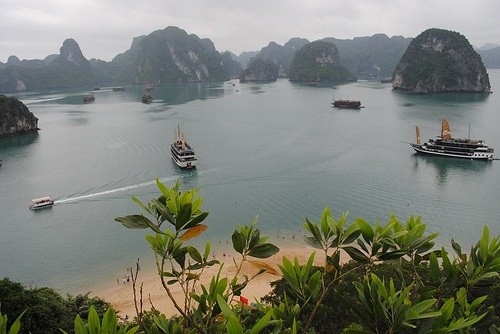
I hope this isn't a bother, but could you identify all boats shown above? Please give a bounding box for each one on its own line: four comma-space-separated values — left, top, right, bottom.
28, 197, 54, 210
410, 137, 494, 161
84, 95, 95, 102
142, 95, 152, 103
171, 126, 197, 169
331, 100, 365, 109
